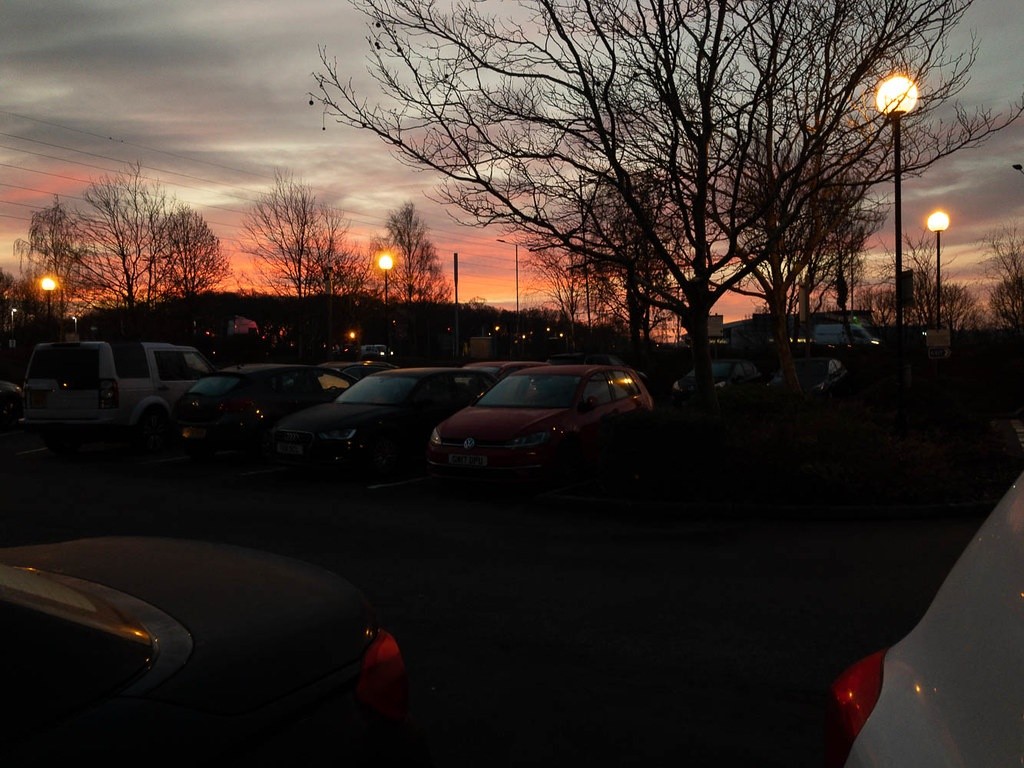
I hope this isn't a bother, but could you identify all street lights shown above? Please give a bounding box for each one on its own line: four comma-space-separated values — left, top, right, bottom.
873, 69, 919, 330
37, 272, 62, 330
375, 247, 403, 359
926, 206, 955, 339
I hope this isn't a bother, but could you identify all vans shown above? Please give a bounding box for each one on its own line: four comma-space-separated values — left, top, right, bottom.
813, 324, 879, 346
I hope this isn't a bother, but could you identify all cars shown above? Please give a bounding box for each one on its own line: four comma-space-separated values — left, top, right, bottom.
824, 469, 1024, 768
269, 369, 500, 486
427, 363, 652, 486
21, 334, 205, 442
3, 533, 430, 768
673, 357, 763, 412
167, 364, 351, 456
